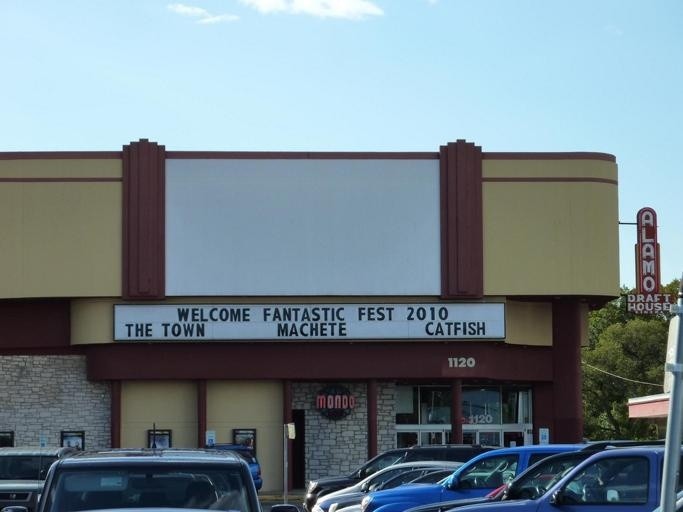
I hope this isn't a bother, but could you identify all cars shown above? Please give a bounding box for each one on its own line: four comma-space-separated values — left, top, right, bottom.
1, 423, 300, 511
